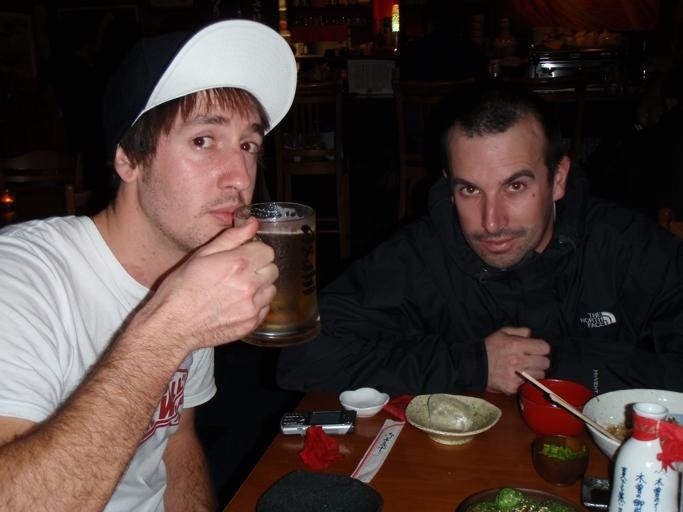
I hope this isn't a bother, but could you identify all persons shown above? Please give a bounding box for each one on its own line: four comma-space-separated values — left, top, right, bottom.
276, 97, 683, 396
334, 15, 683, 222
1, 18, 299, 512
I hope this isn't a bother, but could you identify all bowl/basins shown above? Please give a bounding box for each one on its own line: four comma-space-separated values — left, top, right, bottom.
586, 387, 683, 461
280, 132, 331, 166
338, 387, 390, 420
517, 378, 595, 437
531, 433, 590, 488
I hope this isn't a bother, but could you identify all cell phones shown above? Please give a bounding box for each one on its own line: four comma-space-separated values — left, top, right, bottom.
280, 410, 358, 436
582, 476, 611, 509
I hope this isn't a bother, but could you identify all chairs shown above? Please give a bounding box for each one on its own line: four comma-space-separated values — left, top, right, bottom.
277, 79, 351, 262
391, 77, 475, 220
505, 78, 586, 160
658, 208, 683, 239
0, 85, 113, 229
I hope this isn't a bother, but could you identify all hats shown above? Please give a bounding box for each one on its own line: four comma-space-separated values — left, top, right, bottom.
132, 19, 297, 136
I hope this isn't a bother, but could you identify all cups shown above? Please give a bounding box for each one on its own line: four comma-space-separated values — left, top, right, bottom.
230, 201, 323, 348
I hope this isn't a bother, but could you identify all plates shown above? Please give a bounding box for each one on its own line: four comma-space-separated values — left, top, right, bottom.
454, 488, 583, 511
579, 473, 613, 508
252, 470, 386, 512
405, 393, 502, 446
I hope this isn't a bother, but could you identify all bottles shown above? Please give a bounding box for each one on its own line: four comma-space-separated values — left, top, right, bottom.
541, 27, 619, 48
606, 401, 679, 512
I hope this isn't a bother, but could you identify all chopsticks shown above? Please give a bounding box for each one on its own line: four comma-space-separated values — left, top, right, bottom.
517, 368, 623, 446
